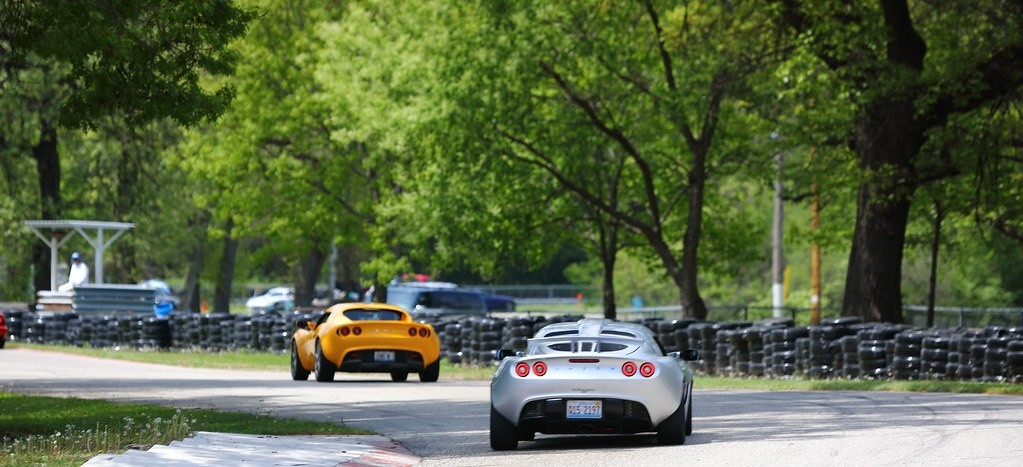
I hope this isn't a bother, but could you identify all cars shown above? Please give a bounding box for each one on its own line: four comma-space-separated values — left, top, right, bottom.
246, 285, 296, 316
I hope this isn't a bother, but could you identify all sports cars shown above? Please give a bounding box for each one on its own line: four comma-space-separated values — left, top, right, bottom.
489, 319, 700, 452
291, 301, 441, 383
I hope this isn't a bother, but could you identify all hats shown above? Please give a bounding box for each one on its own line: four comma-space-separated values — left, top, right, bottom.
71, 252, 81, 260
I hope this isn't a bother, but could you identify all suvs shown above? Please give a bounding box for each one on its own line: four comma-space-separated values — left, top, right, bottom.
362, 279, 516, 313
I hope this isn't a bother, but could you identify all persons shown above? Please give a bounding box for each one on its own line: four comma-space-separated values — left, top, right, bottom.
69, 252, 90, 285
